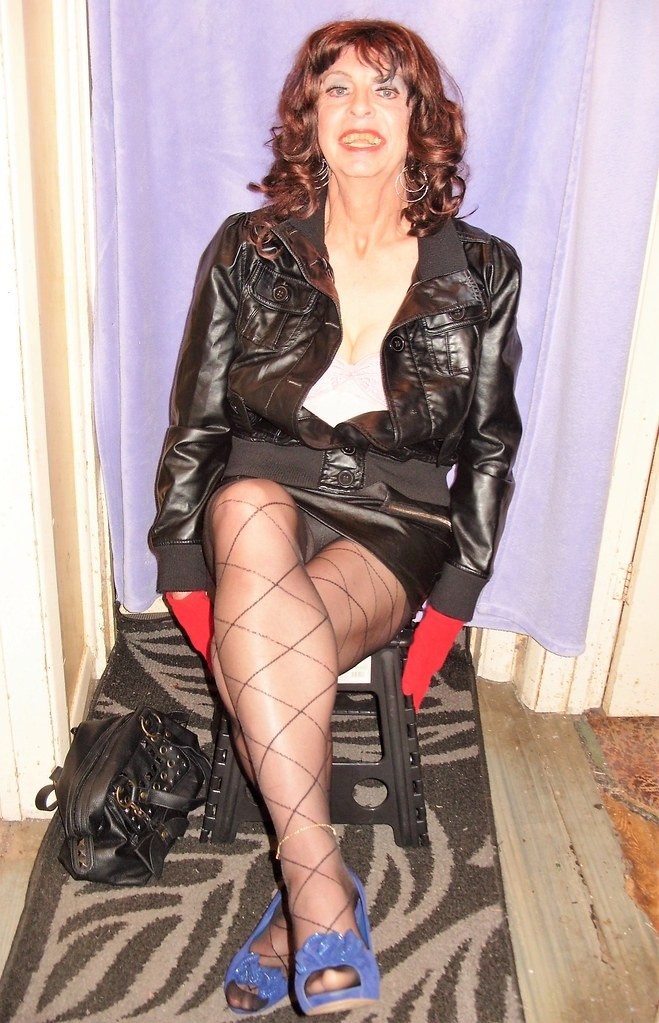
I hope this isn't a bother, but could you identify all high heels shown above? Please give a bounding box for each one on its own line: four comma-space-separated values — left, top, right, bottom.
221, 883, 298, 1019
291, 866, 381, 1017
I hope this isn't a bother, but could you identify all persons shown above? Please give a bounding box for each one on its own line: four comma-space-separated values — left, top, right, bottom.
146, 20, 522, 1015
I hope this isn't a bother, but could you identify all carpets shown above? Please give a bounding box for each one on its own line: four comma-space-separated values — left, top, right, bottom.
0, 599, 526, 1023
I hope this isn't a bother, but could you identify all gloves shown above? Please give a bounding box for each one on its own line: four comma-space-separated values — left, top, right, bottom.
164, 590, 215, 673
401, 605, 465, 715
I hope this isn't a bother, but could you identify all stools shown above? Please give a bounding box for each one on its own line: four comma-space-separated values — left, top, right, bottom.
200, 620, 430, 849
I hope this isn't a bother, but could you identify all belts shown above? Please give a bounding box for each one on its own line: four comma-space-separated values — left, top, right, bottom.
223, 436, 454, 511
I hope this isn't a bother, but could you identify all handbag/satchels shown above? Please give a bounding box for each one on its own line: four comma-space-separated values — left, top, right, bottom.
34, 702, 212, 887
